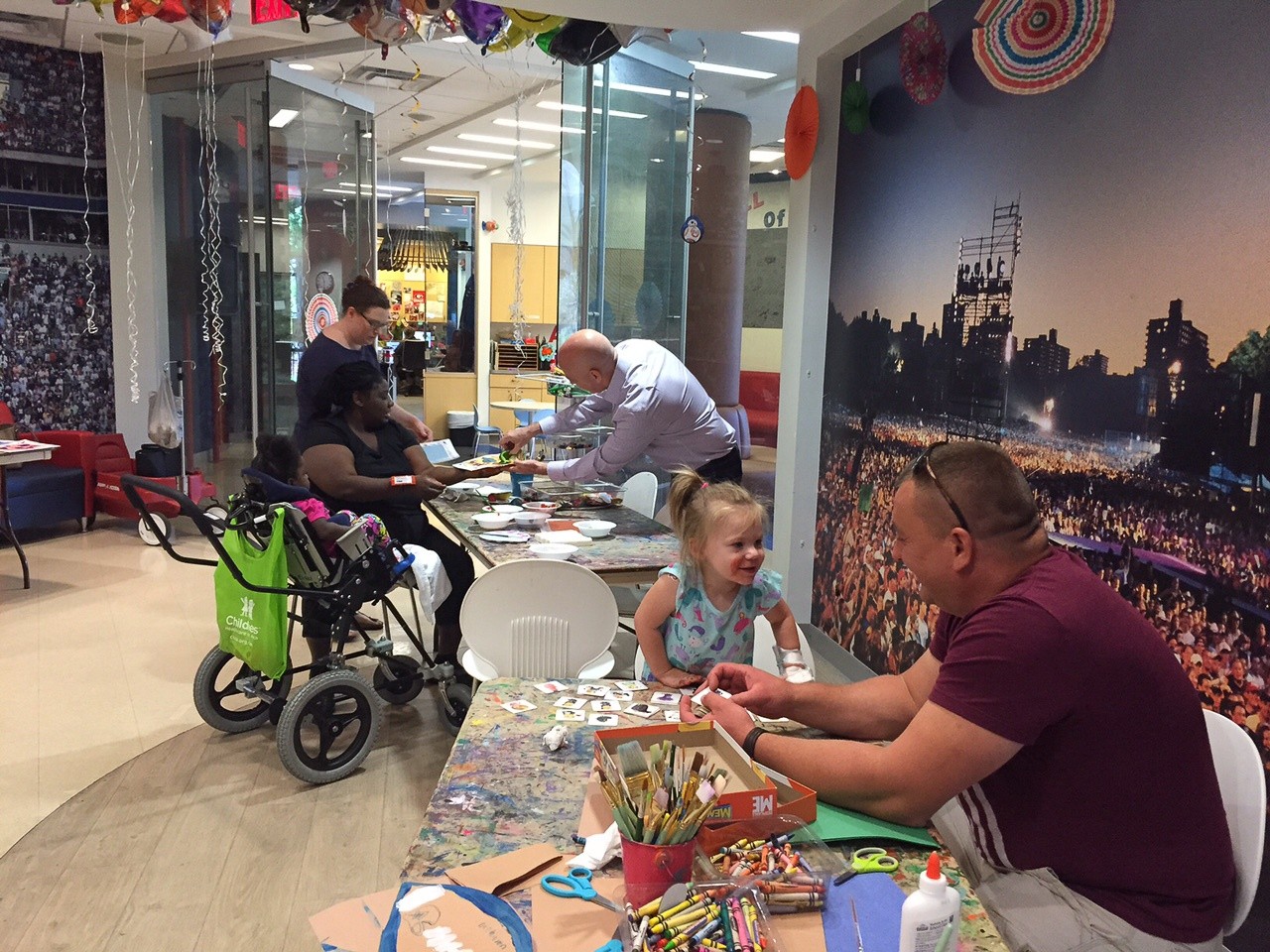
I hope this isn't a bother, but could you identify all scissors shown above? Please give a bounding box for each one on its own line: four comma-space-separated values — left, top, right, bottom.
541, 866, 626, 914
833, 847, 898, 886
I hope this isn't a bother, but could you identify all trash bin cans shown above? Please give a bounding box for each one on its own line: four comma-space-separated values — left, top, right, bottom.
446, 410, 476, 447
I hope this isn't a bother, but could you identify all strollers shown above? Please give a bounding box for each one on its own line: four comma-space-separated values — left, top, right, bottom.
138, 462, 458, 784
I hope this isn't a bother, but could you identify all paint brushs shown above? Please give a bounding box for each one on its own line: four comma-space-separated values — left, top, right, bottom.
592, 738, 732, 845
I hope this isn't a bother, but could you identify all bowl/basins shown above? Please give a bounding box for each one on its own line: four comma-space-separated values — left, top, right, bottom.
573, 519, 616, 537
528, 543, 578, 561
471, 502, 562, 529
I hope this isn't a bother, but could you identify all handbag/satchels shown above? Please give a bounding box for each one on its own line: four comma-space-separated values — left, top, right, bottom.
213, 495, 289, 682
147, 367, 180, 448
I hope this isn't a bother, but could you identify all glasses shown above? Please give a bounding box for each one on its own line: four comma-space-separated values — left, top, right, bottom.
911, 440, 968, 533
358, 310, 385, 330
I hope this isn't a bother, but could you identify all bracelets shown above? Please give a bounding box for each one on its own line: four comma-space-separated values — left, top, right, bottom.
742, 727, 768, 760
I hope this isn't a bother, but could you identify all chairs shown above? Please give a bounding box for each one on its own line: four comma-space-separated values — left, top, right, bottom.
455, 556, 619, 678
0, 399, 97, 534
1201, 703, 1266, 951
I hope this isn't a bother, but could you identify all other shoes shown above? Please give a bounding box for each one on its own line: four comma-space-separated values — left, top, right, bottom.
350, 610, 383, 630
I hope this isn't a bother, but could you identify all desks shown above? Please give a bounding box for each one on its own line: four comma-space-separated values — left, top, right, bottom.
422, 453, 681, 653
371, 677, 1010, 948
1, 438, 55, 591
489, 400, 554, 459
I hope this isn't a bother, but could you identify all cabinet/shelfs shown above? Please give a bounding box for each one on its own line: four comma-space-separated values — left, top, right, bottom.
489, 374, 557, 442
422, 369, 479, 443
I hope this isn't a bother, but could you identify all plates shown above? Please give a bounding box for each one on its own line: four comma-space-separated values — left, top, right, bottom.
480, 529, 529, 544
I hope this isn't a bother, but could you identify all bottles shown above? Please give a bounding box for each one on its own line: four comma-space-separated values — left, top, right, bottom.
899, 850, 959, 952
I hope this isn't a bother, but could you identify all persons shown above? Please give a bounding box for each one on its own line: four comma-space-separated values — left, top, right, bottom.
294, 273, 433, 642
1, 36, 116, 433
814, 406, 1270, 772
632, 465, 815, 688
498, 329, 743, 488
679, 438, 1238, 952
289, 360, 476, 669
250, 431, 432, 589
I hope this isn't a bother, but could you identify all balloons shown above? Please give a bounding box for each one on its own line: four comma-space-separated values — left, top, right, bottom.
280, 0, 673, 66
53, 0, 233, 41
